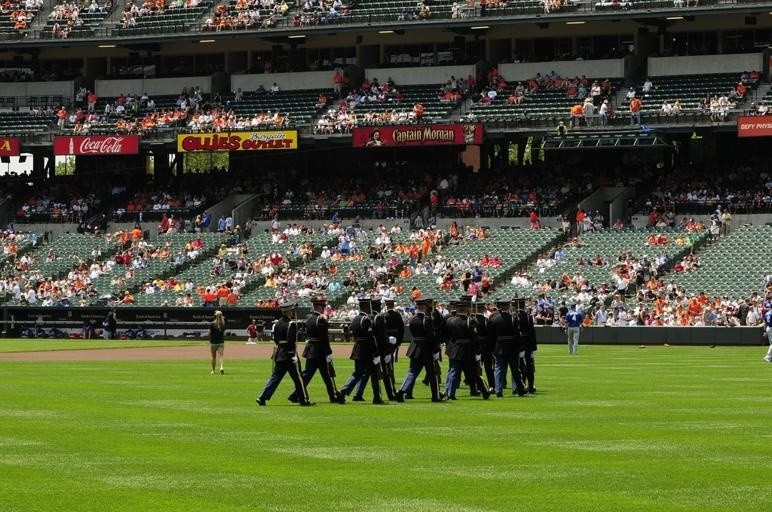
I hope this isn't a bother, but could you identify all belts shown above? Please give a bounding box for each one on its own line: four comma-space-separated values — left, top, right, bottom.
496, 335, 512, 340
456, 338, 470, 343
414, 336, 423, 340
308, 337, 319, 341
356, 336, 366, 341
278, 341, 287, 344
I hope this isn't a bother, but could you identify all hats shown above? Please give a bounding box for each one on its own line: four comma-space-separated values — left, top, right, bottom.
310, 300, 326, 307
498, 297, 524, 307
449, 295, 486, 308
358, 298, 396, 305
279, 302, 296, 312
415, 298, 432, 306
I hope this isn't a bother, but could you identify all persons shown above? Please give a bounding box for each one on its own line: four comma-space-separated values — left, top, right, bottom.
1, 0, 771, 356
209, 309, 225, 375
256, 297, 538, 407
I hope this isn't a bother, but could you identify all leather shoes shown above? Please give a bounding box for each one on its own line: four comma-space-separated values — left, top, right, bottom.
432, 391, 447, 403
472, 385, 535, 400
395, 391, 415, 402
256, 397, 265, 405
352, 395, 364, 401
372, 398, 384, 404
287, 394, 315, 405
330, 390, 346, 404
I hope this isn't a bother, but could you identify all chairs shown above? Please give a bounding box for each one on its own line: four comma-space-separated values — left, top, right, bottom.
1, 0, 772, 327
